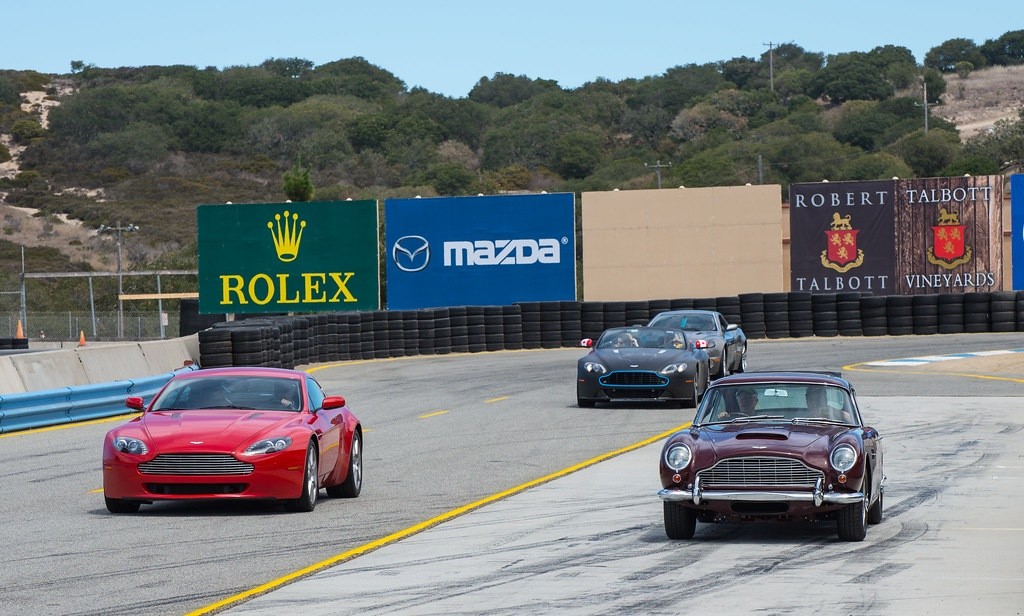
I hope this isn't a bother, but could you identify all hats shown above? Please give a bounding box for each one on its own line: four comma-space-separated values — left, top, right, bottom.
735, 386, 757, 395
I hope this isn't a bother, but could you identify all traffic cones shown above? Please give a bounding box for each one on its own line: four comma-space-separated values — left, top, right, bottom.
78, 330, 85, 346
17, 320, 23, 339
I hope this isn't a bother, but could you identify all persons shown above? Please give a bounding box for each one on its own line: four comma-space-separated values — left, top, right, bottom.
704, 316, 717, 332
806, 386, 853, 424
660, 317, 682, 331
719, 386, 760, 419
272, 380, 299, 412
665, 332, 684, 350
603, 333, 640, 350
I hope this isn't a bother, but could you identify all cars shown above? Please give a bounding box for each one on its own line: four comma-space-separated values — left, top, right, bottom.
631, 310, 747, 378
658, 371, 886, 542
577, 326, 710, 408
102, 367, 363, 512
